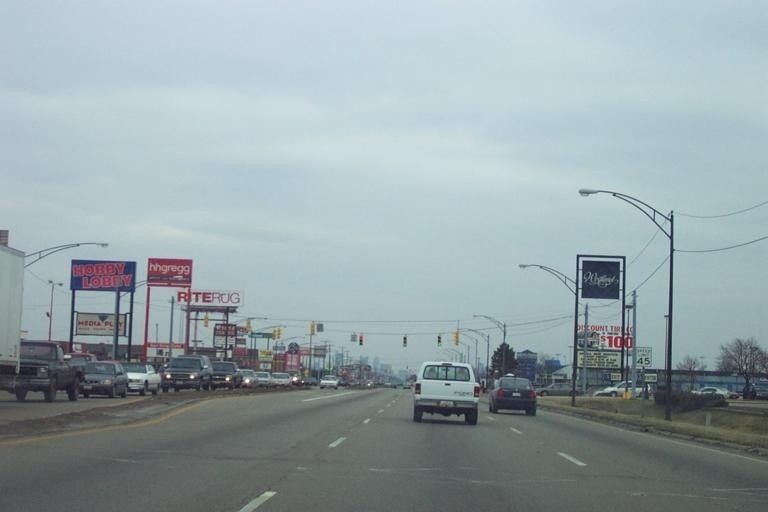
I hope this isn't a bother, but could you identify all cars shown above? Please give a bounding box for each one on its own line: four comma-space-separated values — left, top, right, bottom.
593, 380, 652, 399
690, 386, 767, 400
490, 376, 539, 415
535, 382, 585, 398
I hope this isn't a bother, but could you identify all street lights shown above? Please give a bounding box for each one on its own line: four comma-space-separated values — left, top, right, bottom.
441, 312, 508, 383
663, 314, 669, 380
155, 322, 160, 342
699, 357, 706, 384
578, 187, 676, 423
519, 262, 578, 405
47, 278, 64, 342
23, 242, 110, 275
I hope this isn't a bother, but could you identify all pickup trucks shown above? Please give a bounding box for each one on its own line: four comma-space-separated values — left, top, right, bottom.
414, 361, 481, 428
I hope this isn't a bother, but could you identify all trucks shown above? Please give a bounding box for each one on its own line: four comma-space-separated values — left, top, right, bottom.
0, 242, 24, 391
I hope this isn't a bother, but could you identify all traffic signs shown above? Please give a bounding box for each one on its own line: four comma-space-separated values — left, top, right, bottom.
635, 346, 653, 368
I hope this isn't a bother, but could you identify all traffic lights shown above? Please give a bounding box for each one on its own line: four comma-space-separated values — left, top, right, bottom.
272, 329, 275, 340
402, 336, 407, 347
277, 329, 280, 339
203, 316, 209, 328
360, 336, 362, 345
437, 336, 441, 346
